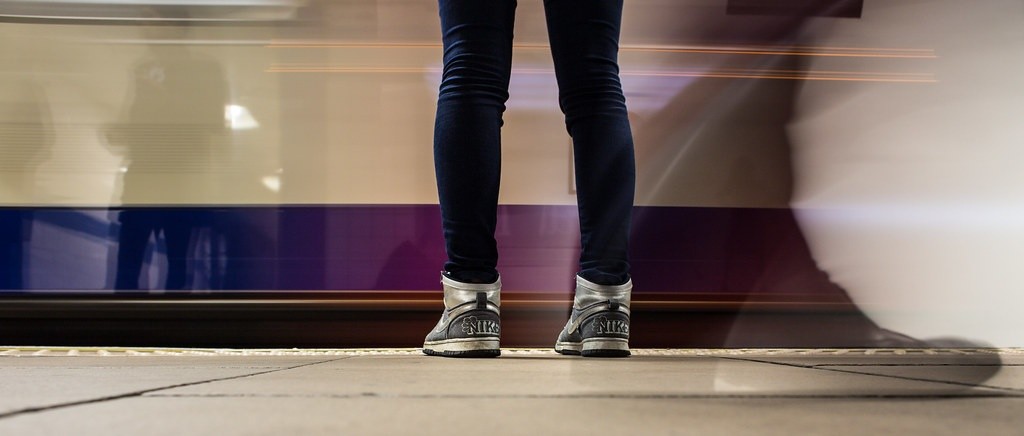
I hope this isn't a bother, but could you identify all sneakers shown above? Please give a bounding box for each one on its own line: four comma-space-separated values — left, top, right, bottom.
423, 271, 501, 357
555, 274, 632, 356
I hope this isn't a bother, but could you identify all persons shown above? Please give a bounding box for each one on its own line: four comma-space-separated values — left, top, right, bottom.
570, 1, 1003, 401
102, 6, 228, 292
422, 0, 636, 357
